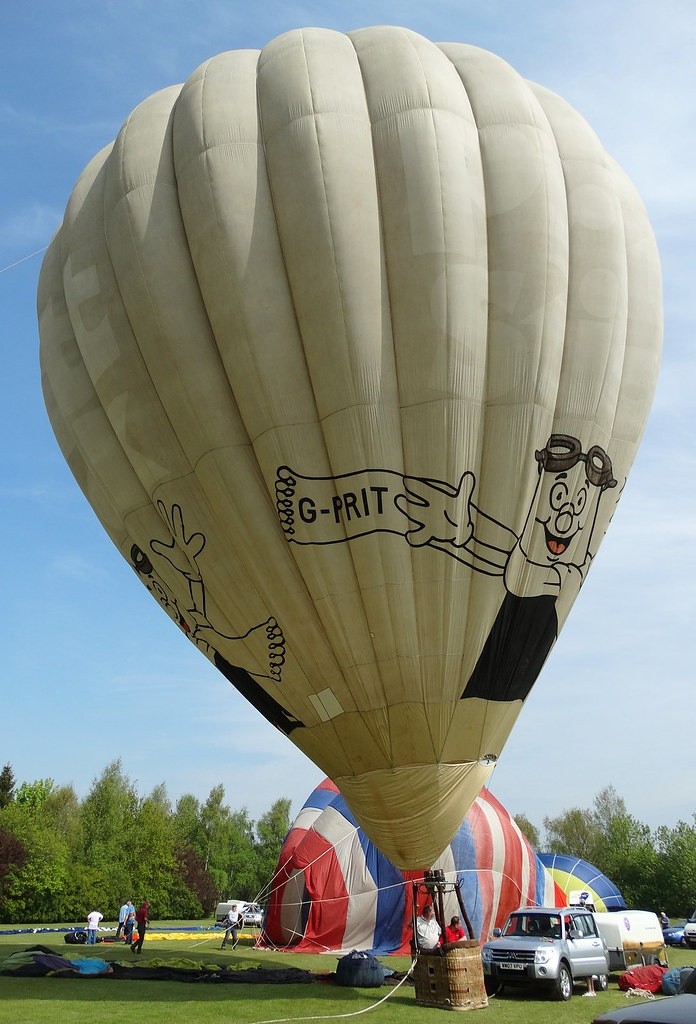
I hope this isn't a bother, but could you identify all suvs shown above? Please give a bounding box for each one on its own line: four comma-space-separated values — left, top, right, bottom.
479, 905, 611, 1001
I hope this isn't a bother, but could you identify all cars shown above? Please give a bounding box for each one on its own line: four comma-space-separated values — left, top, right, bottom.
662, 919, 690, 948
591, 968, 696, 1024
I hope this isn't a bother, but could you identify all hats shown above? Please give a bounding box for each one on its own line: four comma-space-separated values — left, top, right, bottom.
142, 902, 148, 908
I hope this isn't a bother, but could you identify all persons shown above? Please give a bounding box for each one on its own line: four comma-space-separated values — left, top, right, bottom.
115, 899, 131, 937
566, 920, 596, 997
130, 902, 150, 954
437, 916, 467, 949
124, 900, 135, 945
85, 908, 103, 945
660, 912, 670, 931
221, 904, 252, 950
408, 905, 441, 952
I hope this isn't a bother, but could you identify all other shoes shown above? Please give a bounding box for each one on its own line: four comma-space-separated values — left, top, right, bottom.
130, 946, 135, 954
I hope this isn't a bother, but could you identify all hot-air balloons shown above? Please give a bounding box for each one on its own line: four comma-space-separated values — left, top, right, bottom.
36, 24, 668, 1011
535, 852, 628, 913
250, 773, 573, 958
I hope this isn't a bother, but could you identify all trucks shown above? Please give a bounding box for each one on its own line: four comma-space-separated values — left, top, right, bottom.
215, 899, 265, 929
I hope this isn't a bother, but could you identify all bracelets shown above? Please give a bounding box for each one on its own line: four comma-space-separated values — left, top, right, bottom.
146, 920, 148, 921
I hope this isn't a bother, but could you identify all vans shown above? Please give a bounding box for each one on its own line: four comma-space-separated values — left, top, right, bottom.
684, 910, 696, 948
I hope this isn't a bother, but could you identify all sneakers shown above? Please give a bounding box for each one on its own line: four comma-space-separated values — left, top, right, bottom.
582, 992, 596, 996
